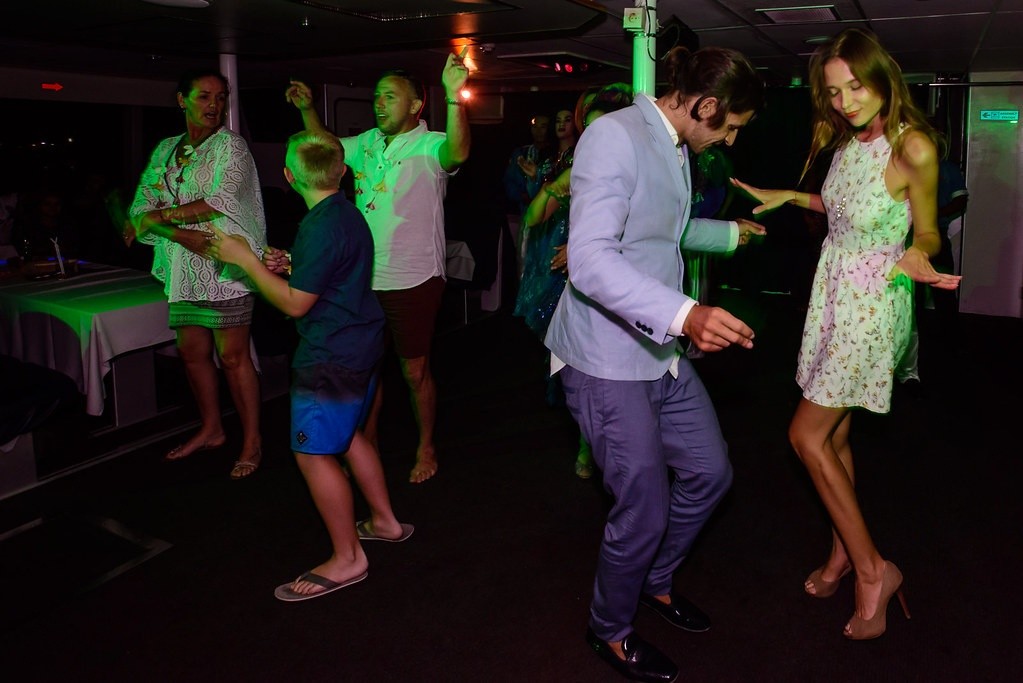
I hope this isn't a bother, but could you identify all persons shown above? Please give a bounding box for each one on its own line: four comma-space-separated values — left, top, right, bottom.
129, 41, 475, 606
513, 86, 973, 480
545, 47, 768, 682
729, 25, 961, 640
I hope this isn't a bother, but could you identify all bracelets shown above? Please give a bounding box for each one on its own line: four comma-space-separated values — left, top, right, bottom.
444, 98, 464, 108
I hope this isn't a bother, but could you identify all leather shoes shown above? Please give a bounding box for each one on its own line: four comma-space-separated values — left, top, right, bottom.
639, 587, 712, 632
586, 625, 680, 683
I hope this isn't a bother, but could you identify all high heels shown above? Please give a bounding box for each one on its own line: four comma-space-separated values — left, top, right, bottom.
843, 560, 911, 640
805, 564, 854, 598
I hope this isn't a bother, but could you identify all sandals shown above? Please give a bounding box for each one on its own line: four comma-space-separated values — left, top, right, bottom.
230, 434, 262, 479
164, 431, 224, 460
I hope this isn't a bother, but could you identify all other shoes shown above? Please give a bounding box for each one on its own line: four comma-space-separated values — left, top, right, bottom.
575, 460, 592, 478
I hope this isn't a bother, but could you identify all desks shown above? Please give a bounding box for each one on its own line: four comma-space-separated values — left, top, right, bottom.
445, 240, 476, 326
0, 258, 181, 439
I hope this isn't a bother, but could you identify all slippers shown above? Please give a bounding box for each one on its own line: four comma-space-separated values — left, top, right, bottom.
356, 521, 414, 542
274, 570, 368, 601
